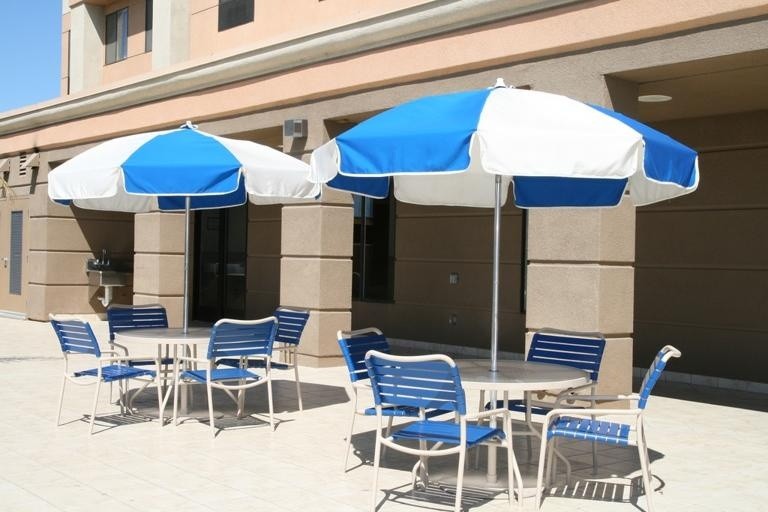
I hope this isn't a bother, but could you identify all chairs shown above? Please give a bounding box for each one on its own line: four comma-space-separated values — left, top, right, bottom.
363, 347, 515, 509
336, 323, 413, 474
47, 299, 311, 436
536, 343, 682, 510
476, 327, 607, 471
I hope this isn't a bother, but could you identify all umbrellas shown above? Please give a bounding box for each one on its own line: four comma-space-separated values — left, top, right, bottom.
46, 119, 322, 413
304, 78, 704, 482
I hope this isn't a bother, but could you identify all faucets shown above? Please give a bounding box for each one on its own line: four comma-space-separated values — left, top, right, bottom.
102, 248, 107, 264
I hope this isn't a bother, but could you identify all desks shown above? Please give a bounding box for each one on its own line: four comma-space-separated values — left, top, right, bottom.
438, 354, 593, 508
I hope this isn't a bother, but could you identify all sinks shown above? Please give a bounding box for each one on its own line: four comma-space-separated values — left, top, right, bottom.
88, 270, 133, 288
